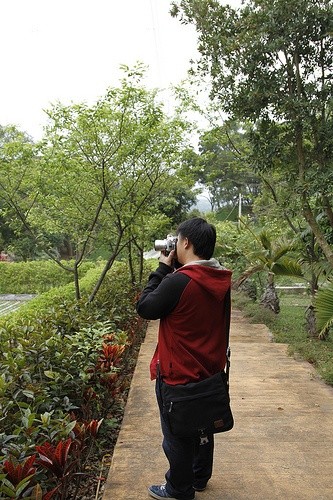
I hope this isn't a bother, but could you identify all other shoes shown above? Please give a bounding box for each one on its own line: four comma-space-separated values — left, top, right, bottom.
148, 481, 178, 500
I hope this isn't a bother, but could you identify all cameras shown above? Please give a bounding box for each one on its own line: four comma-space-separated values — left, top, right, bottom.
154, 235, 175, 252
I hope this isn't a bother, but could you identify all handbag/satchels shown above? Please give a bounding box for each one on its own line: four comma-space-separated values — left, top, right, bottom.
157, 370, 234, 439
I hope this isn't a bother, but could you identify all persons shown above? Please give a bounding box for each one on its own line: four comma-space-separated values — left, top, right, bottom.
136, 218, 233, 500
